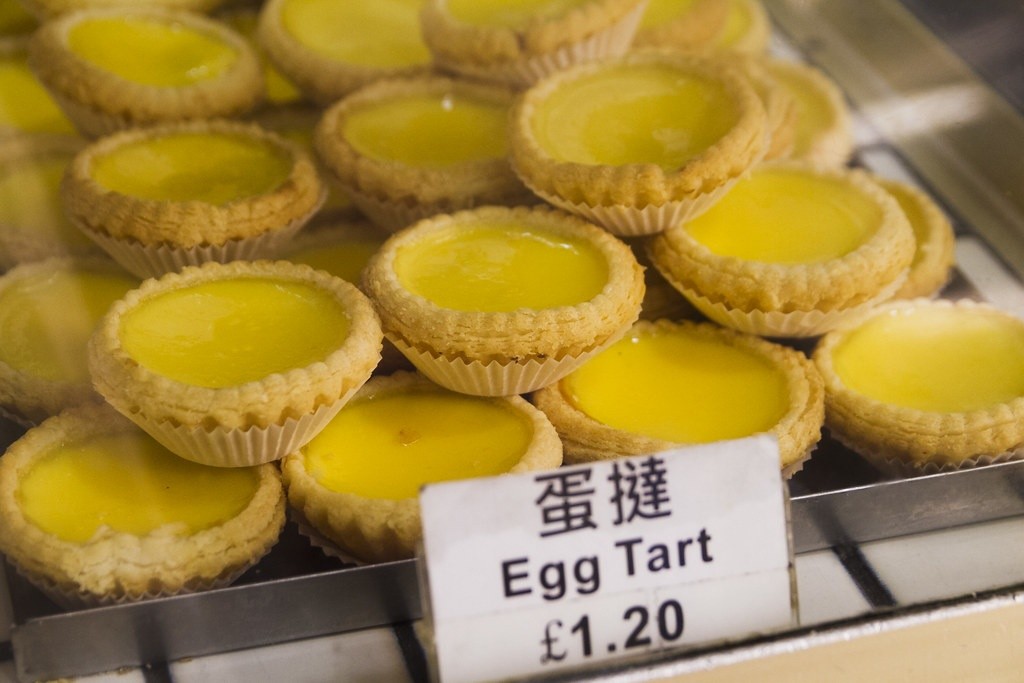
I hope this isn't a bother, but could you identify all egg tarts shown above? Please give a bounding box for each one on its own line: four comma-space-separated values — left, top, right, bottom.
0, 1, 1024, 606
